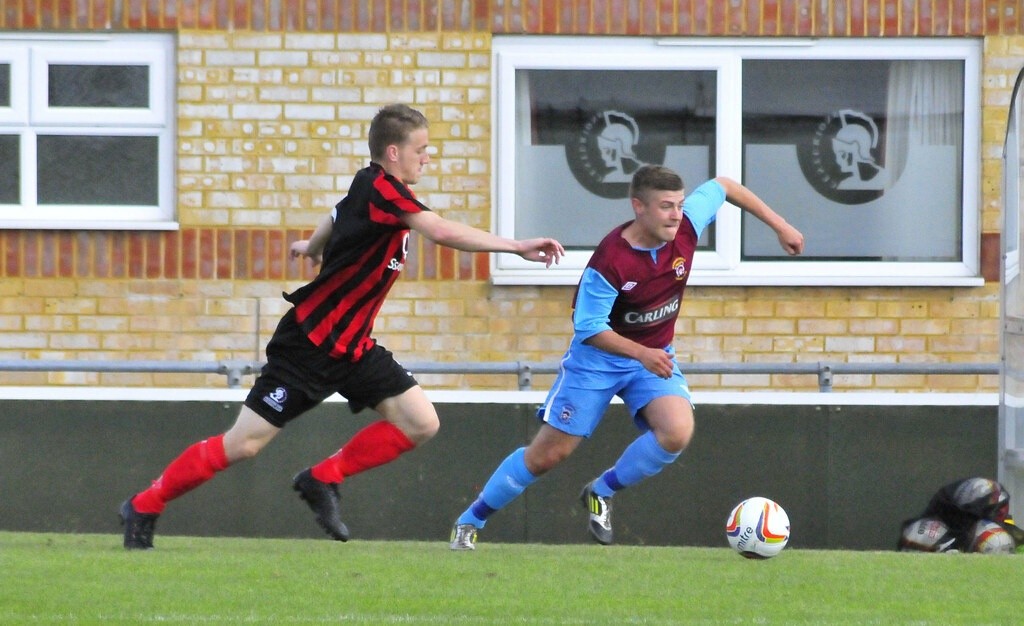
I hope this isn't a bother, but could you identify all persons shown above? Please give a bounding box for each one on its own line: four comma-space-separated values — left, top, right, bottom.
449, 165, 804, 552
119, 103, 566, 550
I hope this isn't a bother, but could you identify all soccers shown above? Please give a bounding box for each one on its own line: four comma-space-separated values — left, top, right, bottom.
898, 477, 1015, 553
725, 496, 792, 561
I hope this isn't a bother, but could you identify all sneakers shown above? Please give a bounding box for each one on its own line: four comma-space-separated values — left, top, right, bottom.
580, 481, 615, 546
449, 518, 478, 550
292, 466, 349, 542
117, 494, 161, 550
